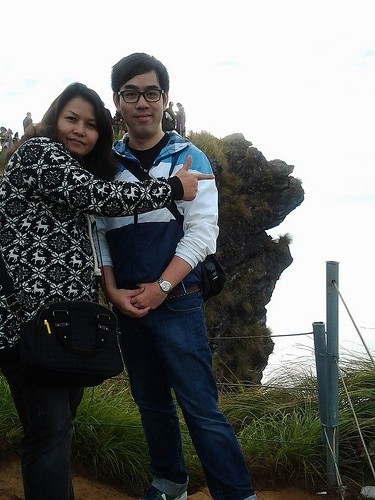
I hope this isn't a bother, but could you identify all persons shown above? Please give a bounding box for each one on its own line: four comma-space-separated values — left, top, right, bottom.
88, 53, 257, 500
0, 102, 187, 151
0, 82, 215, 500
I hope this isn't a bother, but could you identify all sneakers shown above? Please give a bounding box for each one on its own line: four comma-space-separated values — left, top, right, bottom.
137, 485, 187, 500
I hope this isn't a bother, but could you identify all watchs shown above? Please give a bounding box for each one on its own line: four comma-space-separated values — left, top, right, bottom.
154, 276, 174, 294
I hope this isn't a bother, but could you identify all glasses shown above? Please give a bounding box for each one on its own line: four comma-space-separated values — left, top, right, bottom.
117, 89, 165, 103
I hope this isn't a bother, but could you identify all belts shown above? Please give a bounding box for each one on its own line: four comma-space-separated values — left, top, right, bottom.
166, 283, 200, 299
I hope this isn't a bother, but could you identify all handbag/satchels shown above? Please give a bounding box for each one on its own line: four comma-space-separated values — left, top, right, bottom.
19, 301, 124, 387
201, 254, 226, 302
